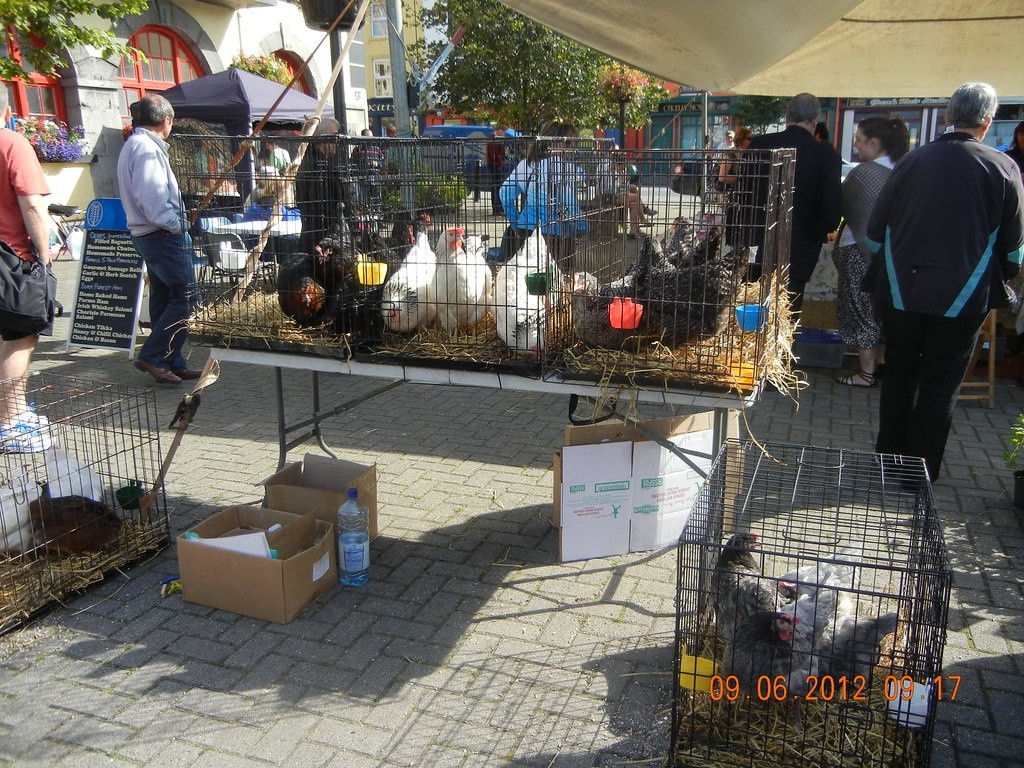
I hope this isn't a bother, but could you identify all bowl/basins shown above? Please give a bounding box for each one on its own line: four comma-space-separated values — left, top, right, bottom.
220, 242, 246, 269
697, 230, 708, 238
608, 297, 643, 329
357, 254, 387, 285
680, 655, 718, 692
741, 246, 758, 264
735, 302, 769, 332
888, 680, 928, 728
525, 265, 553, 295
116, 486, 143, 510
488, 248, 505, 262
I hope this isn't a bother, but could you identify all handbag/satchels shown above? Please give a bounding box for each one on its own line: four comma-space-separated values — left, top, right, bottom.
711, 154, 735, 193
501, 224, 515, 258
0, 241, 55, 334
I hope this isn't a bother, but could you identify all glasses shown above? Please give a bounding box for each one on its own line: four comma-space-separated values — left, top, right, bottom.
743, 137, 753, 142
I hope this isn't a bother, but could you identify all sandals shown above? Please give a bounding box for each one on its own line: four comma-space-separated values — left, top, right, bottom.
837, 369, 876, 388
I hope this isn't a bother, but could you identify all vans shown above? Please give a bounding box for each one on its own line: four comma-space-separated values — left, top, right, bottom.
421, 126, 585, 191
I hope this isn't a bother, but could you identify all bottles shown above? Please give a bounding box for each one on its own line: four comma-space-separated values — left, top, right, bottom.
49, 228, 56, 245
338, 489, 370, 587
268, 524, 282, 535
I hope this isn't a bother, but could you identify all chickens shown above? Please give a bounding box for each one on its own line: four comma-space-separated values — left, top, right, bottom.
0, 444, 124, 563
277, 212, 751, 356
710, 531, 902, 725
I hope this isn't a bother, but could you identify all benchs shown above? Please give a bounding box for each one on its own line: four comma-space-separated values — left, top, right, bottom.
580, 198, 622, 236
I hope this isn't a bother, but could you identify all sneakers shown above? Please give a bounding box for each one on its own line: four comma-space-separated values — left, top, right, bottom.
18, 411, 50, 432
0, 422, 57, 454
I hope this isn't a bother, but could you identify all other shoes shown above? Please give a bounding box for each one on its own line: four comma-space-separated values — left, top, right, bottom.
492, 209, 506, 216
643, 206, 658, 216
377, 221, 388, 229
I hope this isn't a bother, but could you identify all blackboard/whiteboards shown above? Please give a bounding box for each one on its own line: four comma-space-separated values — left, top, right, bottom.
66, 230, 145, 352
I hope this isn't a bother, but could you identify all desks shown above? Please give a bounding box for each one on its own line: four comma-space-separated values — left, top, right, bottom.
47, 213, 92, 260
218, 220, 303, 286
210, 347, 768, 612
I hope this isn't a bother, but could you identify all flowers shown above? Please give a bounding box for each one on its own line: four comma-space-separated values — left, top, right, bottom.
232, 54, 295, 87
603, 69, 643, 101
15, 114, 83, 162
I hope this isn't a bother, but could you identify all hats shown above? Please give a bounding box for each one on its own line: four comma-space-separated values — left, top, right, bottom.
301, 117, 341, 136
726, 130, 735, 139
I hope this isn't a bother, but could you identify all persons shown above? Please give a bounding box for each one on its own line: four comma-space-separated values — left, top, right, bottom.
255, 141, 291, 185
350, 128, 386, 227
294, 117, 341, 251
719, 128, 753, 183
861, 81, 1024, 494
486, 129, 505, 215
499, 121, 588, 273
994, 137, 1009, 152
117, 95, 203, 384
741, 92, 842, 323
831, 117, 911, 387
1004, 121, 1024, 173
0, 81, 55, 452
713, 130, 735, 164
597, 145, 658, 239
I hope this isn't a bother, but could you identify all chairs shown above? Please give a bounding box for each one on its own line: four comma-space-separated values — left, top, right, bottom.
197, 218, 276, 289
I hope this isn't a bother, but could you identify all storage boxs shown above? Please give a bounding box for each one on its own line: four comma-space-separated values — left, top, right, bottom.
176, 507, 335, 624
795, 340, 849, 368
554, 410, 744, 562
253, 452, 378, 547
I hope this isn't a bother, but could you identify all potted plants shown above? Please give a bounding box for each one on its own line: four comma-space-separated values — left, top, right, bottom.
1002, 413, 1024, 512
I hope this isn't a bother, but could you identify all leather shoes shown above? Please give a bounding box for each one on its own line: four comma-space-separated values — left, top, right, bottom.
170, 367, 203, 379
134, 356, 183, 384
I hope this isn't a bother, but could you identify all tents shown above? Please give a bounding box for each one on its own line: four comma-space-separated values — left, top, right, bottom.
130, 64, 335, 196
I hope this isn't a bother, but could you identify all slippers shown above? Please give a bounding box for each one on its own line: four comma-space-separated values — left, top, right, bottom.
628, 232, 646, 239
638, 223, 654, 227
